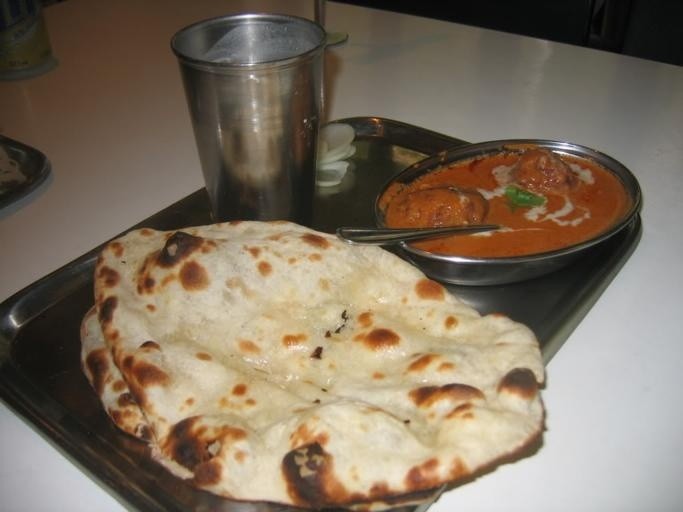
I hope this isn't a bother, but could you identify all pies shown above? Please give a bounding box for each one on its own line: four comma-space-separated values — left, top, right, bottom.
91, 217, 548, 505
79, 303, 444, 512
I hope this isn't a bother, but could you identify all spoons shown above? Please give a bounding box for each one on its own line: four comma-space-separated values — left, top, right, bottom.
316, 121, 360, 190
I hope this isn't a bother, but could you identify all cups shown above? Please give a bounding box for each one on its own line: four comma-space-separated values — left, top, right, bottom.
0, 0, 57, 83
170, 13, 348, 228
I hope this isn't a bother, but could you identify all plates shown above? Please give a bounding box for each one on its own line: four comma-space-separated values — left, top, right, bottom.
372, 139, 642, 287
